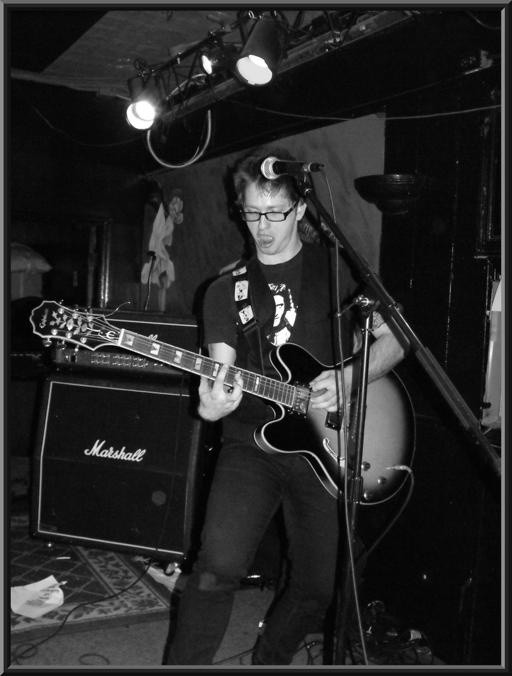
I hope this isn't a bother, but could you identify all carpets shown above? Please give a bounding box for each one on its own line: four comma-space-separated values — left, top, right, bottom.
8, 494, 173, 645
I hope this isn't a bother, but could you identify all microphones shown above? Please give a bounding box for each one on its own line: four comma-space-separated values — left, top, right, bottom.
260, 156, 324, 181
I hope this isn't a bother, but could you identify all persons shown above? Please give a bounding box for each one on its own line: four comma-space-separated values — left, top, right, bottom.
140, 196, 184, 315
166, 151, 411, 664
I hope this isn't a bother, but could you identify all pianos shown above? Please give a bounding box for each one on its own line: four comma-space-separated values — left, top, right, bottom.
47, 308, 204, 377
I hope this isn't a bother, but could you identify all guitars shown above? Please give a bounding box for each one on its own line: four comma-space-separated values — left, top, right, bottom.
29, 300, 416, 506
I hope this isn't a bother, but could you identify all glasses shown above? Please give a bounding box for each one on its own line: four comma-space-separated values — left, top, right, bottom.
239, 202, 300, 223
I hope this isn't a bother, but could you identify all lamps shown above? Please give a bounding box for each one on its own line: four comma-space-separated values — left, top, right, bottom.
198, 43, 239, 75
125, 80, 162, 130
236, 10, 289, 87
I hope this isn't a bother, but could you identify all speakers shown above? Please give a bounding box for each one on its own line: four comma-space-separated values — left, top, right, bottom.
30, 377, 204, 577
